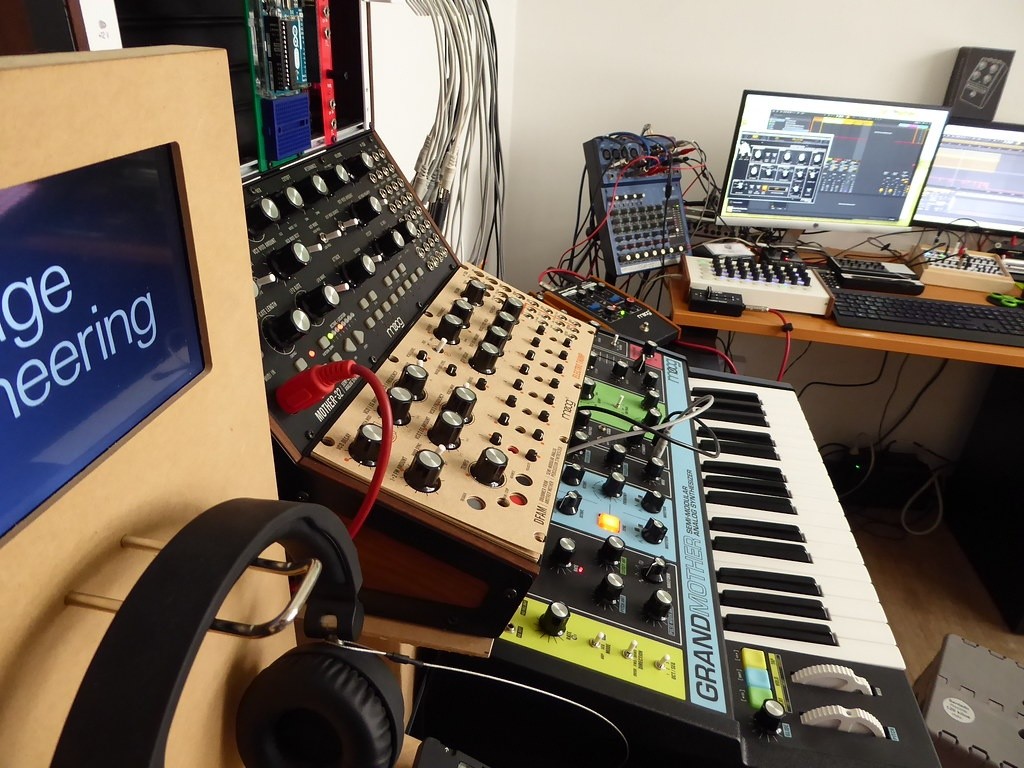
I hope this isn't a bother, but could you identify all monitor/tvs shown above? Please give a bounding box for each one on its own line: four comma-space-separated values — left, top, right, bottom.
714, 89, 953, 263
908, 115, 1024, 240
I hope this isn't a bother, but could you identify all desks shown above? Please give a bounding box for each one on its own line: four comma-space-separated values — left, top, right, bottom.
665, 241, 1024, 372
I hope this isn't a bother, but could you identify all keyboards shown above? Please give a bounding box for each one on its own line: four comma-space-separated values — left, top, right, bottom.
829, 287, 1024, 348
